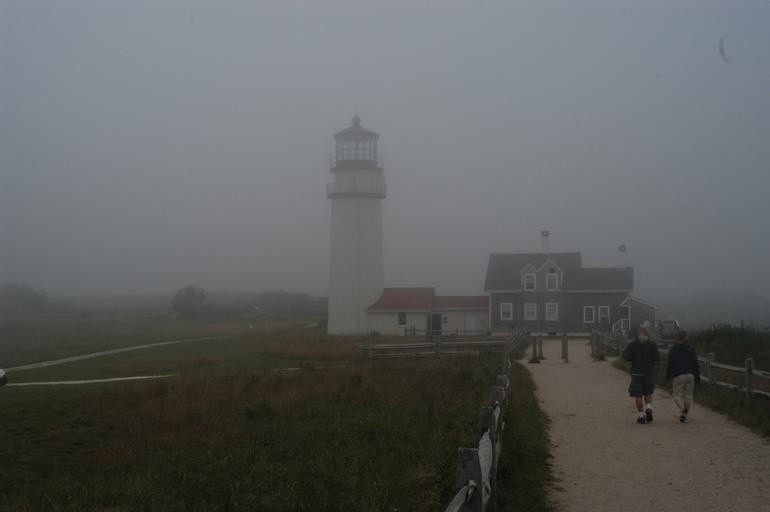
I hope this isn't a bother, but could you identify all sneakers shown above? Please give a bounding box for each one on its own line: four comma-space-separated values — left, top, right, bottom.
637, 409, 652, 424
680, 417, 688, 423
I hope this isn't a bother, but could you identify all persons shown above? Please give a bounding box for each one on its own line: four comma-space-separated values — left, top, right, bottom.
667, 331, 701, 421
623, 320, 661, 422
